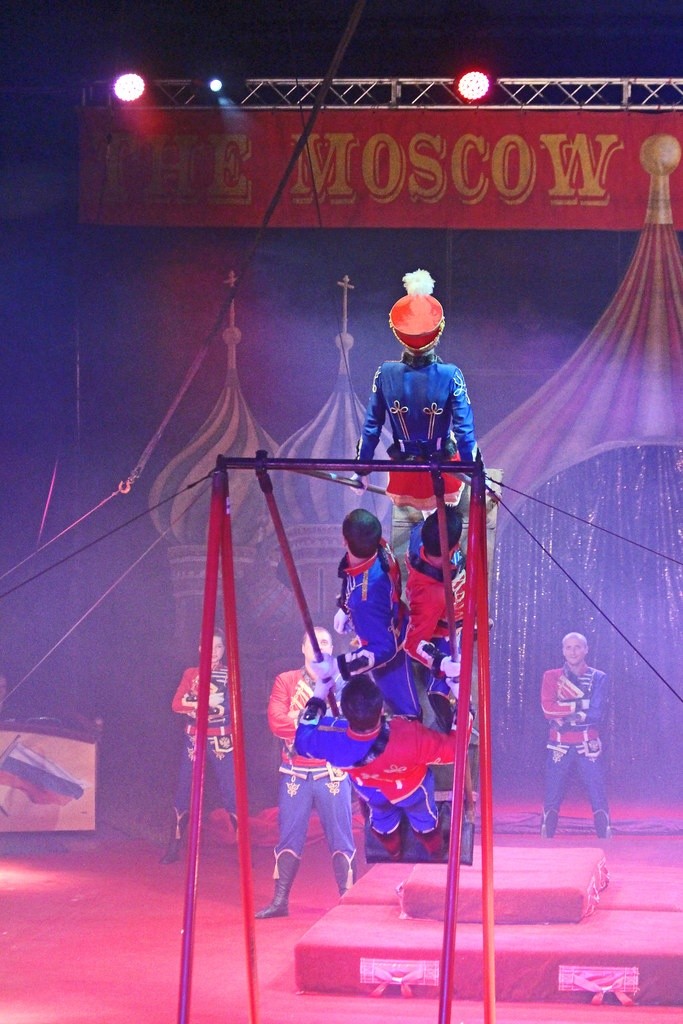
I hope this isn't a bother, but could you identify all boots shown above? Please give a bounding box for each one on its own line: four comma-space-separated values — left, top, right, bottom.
370, 817, 406, 862
411, 802, 451, 861
330, 849, 359, 898
157, 806, 188, 865
228, 811, 237, 844
591, 809, 611, 838
540, 807, 559, 839
254, 845, 302, 919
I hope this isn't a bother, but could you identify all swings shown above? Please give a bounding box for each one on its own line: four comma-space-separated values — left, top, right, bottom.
249, 450, 504, 867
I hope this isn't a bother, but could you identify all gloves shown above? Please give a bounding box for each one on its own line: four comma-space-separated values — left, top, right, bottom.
310, 653, 339, 678
445, 678, 472, 704
348, 473, 368, 496
313, 678, 335, 700
333, 607, 354, 633
208, 693, 224, 705
440, 655, 461, 678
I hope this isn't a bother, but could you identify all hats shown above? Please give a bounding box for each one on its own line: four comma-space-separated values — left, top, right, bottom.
389, 269, 446, 354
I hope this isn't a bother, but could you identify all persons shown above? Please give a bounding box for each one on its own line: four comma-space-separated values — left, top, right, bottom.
0, 674, 41, 725
349, 270, 503, 529
254, 503, 494, 920
540, 631, 614, 840
157, 625, 256, 868
